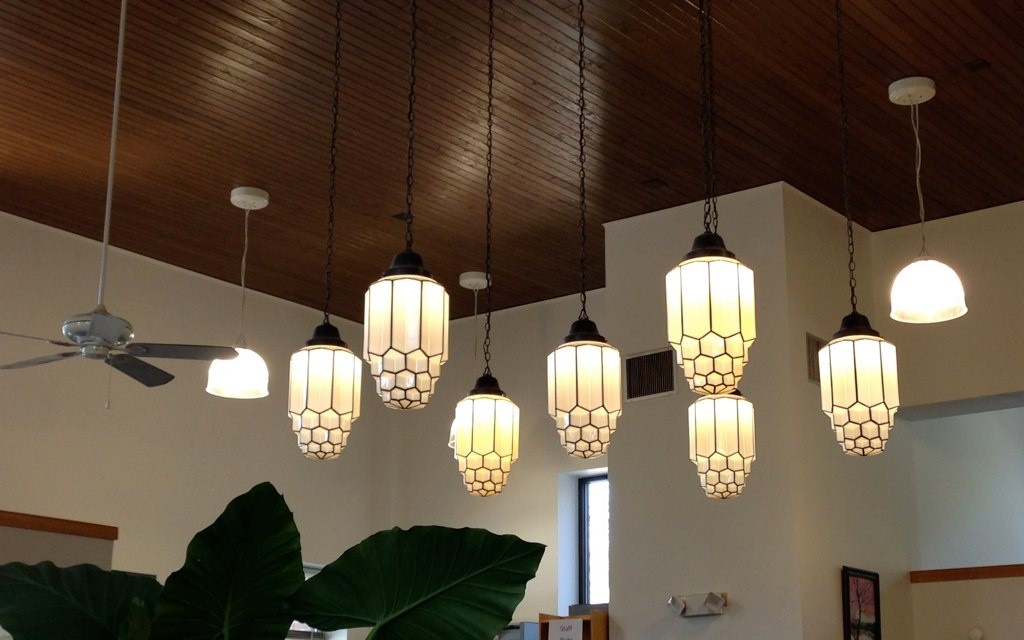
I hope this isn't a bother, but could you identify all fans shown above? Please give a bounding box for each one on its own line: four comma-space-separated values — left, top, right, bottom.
0, 0, 239, 411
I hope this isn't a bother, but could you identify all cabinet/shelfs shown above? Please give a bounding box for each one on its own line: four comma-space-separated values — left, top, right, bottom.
497, 603, 609, 640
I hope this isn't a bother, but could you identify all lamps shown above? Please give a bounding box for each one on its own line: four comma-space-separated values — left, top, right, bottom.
448, 270, 492, 449
687, 0, 754, 500
205, 185, 272, 401
548, 0, 623, 461
288, 0, 362, 460
886, 73, 972, 328
454, 0, 521, 496
818, 0, 901, 461
362, 0, 451, 411
668, 592, 726, 618
665, 0, 755, 393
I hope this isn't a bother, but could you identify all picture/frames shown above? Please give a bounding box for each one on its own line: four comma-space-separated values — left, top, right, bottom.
840, 565, 882, 640
